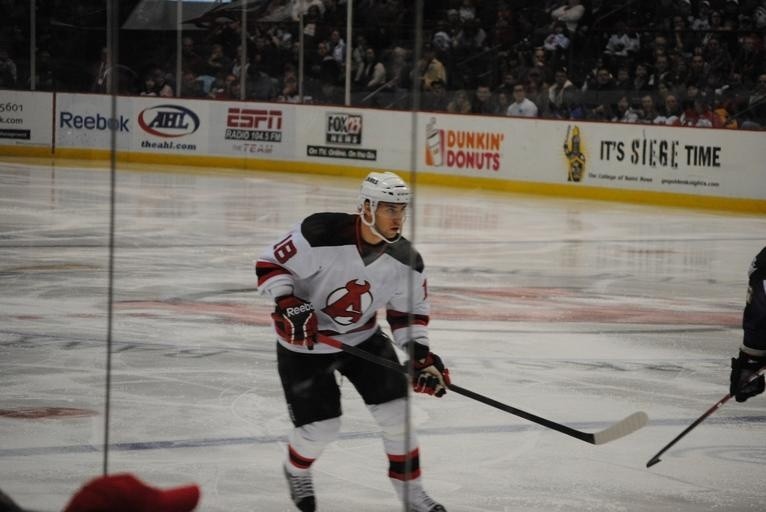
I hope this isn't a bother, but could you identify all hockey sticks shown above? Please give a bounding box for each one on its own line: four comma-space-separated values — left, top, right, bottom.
313, 334, 648, 445
647, 369, 766, 466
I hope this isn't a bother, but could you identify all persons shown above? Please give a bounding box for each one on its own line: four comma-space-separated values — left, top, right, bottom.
254, 169, 451, 511
1, 1, 766, 130
728, 248, 766, 402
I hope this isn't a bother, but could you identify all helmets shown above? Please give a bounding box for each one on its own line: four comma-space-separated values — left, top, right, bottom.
358, 169, 411, 211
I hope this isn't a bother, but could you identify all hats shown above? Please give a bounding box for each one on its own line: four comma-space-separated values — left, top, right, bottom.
58, 472, 201, 511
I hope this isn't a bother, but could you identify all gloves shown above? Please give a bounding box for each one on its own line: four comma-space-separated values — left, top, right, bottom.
402, 352, 452, 399
269, 293, 319, 352
728, 351, 765, 402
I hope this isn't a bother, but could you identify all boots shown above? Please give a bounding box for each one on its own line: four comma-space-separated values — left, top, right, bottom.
387, 468, 449, 512
281, 460, 317, 512
